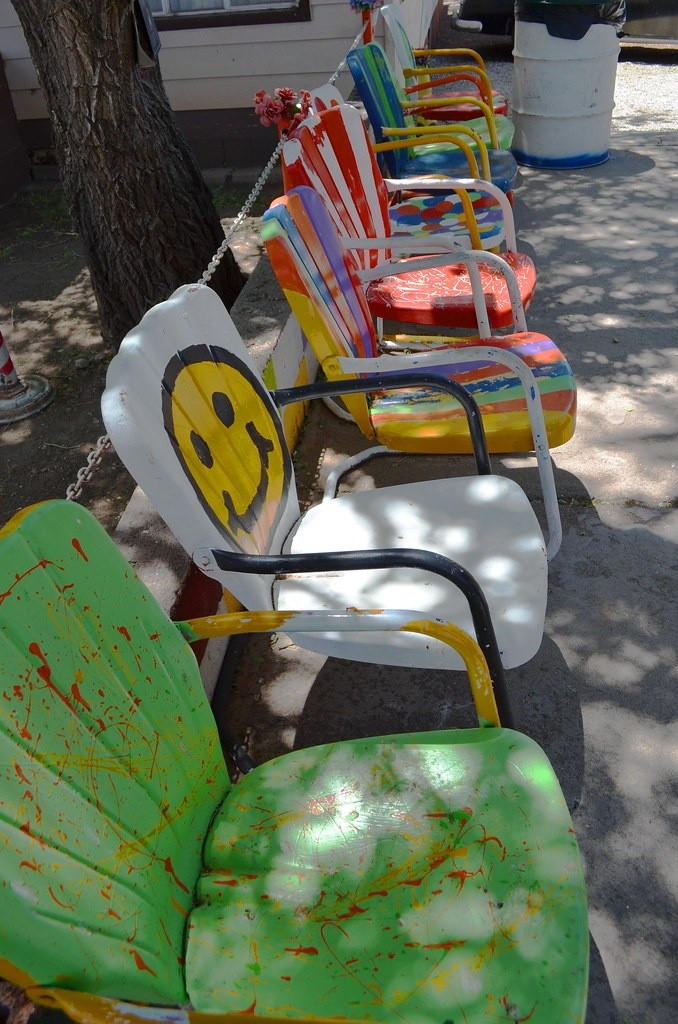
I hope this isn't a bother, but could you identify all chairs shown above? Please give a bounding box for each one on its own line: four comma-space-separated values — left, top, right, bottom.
346, 40, 518, 204
101, 280, 551, 726
302, 83, 517, 252
282, 103, 538, 337
0, 498, 594, 1024
256, 186, 576, 471
379, 7, 508, 118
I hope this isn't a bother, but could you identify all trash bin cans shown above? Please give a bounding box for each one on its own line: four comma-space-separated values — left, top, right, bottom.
511, 0, 626, 170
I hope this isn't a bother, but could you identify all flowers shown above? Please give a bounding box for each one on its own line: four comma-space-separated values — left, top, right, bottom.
252, 82, 311, 134
350, 0, 384, 14
